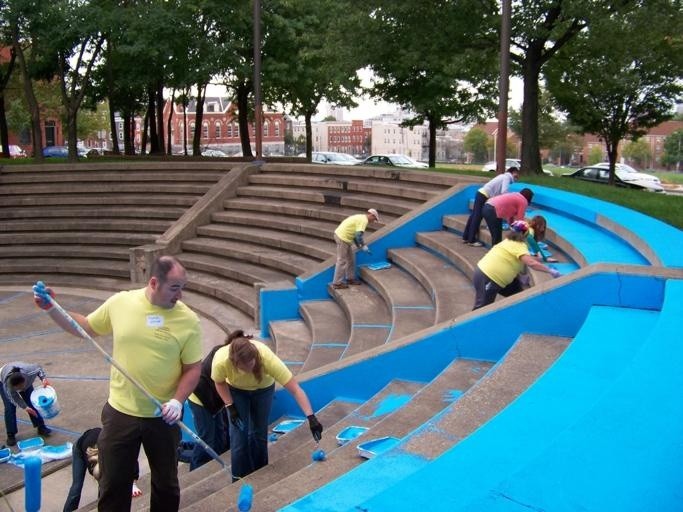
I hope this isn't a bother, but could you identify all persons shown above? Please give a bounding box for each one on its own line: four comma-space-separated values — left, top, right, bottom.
32, 254, 203, 512
481, 187, 534, 246
332, 208, 379, 289
0, 361, 50, 446
462, 166, 521, 246
210, 338, 323, 484
189, 329, 256, 471
473, 221, 563, 309
524, 214, 558, 263
62, 428, 143, 512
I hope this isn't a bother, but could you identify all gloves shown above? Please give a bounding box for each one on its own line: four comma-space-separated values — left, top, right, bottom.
153, 399, 183, 425
42, 378, 52, 388
362, 245, 368, 251
32, 280, 56, 310
550, 270, 560, 279
307, 413, 323, 443
132, 483, 143, 497
25, 407, 38, 418
226, 404, 244, 429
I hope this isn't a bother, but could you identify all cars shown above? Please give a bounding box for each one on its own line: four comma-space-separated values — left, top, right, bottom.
39, 147, 83, 158
484, 158, 552, 179
2, 144, 27, 158
300, 150, 429, 169
81, 144, 271, 155
561, 162, 666, 197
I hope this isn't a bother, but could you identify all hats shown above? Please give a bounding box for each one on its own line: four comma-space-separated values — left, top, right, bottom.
368, 208, 378, 221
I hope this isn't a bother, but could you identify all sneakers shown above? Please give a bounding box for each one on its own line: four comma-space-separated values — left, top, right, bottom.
7, 436, 16, 446
38, 425, 51, 435
347, 280, 361, 285
332, 284, 349, 289
467, 242, 484, 247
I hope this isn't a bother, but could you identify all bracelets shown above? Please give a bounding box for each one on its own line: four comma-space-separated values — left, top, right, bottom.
224, 402, 233, 410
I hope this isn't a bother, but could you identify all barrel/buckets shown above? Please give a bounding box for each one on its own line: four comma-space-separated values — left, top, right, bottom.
29, 384, 61, 421
